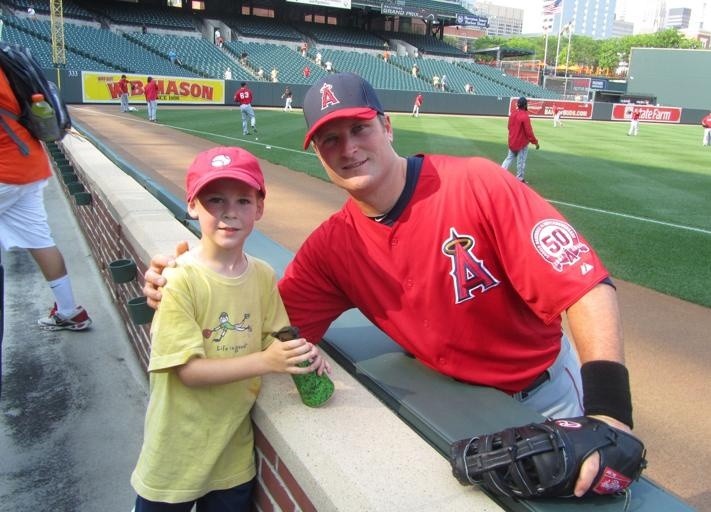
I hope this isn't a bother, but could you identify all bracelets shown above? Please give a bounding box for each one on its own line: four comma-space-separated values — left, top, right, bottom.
581, 360, 634, 429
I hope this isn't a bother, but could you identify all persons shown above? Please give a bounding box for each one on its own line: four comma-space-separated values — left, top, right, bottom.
169, 48, 177, 65
281, 86, 293, 112
701, 112, 711, 146
552, 103, 563, 128
142, 22, 147, 34
233, 81, 257, 136
215, 28, 332, 82
0, 41, 93, 330
384, 41, 475, 94
129, 146, 333, 512
117, 75, 131, 112
144, 77, 159, 122
502, 97, 539, 184
142, 72, 646, 500
625, 108, 641, 136
28, 5, 36, 21
412, 92, 423, 118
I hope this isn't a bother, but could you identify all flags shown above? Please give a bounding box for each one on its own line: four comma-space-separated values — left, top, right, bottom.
560, 16, 575, 36
541, 16, 554, 31
543, 0, 561, 14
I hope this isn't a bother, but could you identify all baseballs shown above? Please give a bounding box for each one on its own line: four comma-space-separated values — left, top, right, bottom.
255, 138, 258, 141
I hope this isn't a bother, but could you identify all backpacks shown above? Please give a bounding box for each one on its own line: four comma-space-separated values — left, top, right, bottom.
0, 42, 71, 155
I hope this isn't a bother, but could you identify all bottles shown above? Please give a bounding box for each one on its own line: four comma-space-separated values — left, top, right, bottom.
30, 93, 61, 142
271, 326, 336, 408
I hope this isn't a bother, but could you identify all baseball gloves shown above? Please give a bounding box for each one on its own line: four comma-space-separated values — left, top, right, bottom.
448, 414, 648, 502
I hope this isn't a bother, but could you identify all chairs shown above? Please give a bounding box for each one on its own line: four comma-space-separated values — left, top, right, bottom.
0, 0, 574, 100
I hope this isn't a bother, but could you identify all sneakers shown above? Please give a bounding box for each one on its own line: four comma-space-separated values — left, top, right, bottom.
37, 300, 92, 330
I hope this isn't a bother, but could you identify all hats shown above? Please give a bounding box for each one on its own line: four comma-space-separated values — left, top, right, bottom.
303, 72, 384, 151
518, 97, 529, 106
186, 148, 266, 203
